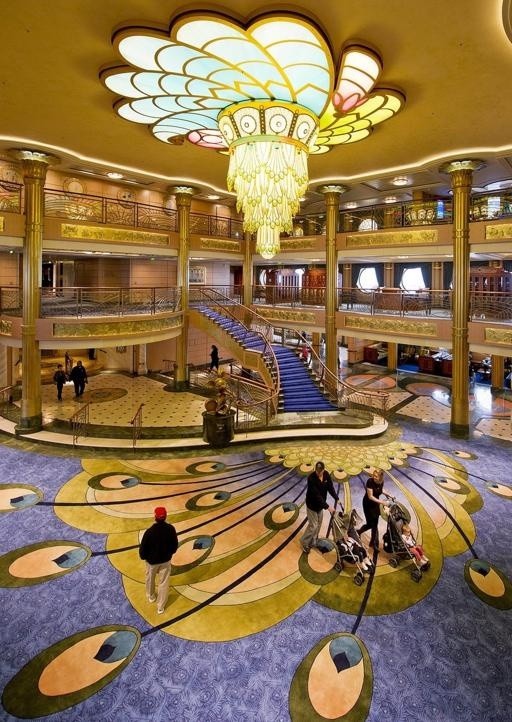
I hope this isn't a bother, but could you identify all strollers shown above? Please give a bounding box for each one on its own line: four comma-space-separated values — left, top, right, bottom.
382, 497, 431, 584
328, 502, 376, 588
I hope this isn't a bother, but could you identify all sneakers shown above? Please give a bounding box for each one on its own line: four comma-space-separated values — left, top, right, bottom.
303, 542, 312, 553
157, 607, 164, 615
148, 597, 156, 603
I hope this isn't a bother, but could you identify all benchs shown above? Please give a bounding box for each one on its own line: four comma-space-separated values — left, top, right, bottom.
420, 349, 452, 375
364, 342, 388, 363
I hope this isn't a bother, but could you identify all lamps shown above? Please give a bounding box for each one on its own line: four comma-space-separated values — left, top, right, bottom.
215, 101, 323, 262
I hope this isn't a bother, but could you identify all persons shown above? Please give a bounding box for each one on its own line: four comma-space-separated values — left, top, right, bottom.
356, 469, 392, 547
468, 354, 472, 377
301, 331, 306, 345
401, 525, 430, 565
341, 529, 373, 571
320, 338, 324, 358
65, 352, 71, 374
482, 354, 492, 373
209, 345, 218, 372
298, 462, 342, 554
69, 361, 87, 398
139, 507, 178, 614
54, 365, 68, 400
337, 341, 340, 369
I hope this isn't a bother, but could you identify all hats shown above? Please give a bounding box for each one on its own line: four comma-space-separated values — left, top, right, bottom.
154, 507, 167, 520
315, 462, 324, 471
